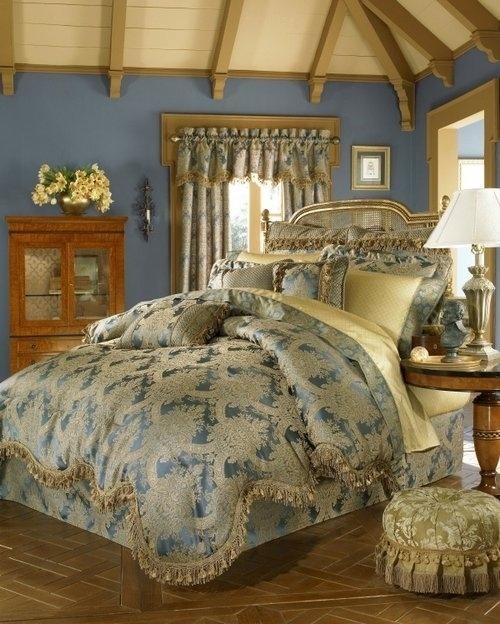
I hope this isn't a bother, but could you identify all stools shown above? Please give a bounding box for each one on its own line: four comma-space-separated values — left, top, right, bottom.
375, 486, 500, 595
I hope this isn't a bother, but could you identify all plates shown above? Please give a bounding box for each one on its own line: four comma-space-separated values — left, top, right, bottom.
409, 355, 481, 367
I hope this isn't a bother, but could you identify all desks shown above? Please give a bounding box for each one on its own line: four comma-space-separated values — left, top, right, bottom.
400, 356, 500, 498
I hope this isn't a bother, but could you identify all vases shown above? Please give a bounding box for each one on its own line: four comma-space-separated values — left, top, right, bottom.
58, 194, 92, 215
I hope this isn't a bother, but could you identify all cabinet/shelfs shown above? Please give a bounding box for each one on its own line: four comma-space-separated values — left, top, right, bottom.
9, 338, 86, 374
10, 233, 124, 337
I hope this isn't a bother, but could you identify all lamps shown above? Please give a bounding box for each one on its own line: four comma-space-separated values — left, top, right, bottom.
423, 188, 500, 362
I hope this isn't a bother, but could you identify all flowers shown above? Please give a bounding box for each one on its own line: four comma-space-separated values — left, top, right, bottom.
30, 161, 116, 216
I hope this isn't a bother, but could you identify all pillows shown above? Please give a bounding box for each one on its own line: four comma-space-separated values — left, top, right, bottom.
273, 255, 349, 310
213, 258, 294, 291
226, 250, 324, 263
348, 224, 453, 295
264, 223, 350, 253
348, 250, 452, 338
345, 270, 449, 358
112, 299, 231, 348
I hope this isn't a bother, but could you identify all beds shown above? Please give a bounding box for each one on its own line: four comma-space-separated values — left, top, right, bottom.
0, 194, 472, 614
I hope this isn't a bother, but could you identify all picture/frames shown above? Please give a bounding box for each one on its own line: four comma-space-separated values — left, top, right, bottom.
351, 145, 391, 191
